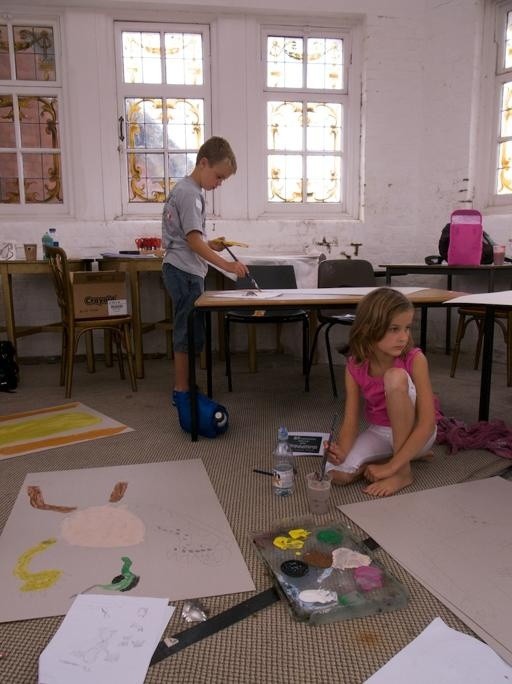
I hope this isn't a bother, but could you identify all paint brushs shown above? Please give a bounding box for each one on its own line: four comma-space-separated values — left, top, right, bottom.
319, 412, 338, 481
221, 242, 262, 293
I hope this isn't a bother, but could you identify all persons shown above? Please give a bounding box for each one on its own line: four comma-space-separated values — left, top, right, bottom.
323, 287, 441, 497
163, 136, 251, 405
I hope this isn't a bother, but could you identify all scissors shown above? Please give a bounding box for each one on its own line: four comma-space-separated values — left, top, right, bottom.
135, 237, 161, 252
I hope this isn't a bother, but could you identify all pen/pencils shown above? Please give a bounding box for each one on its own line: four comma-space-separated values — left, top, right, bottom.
254, 470, 274, 476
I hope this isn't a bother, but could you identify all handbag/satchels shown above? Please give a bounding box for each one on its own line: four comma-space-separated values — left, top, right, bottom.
175, 391, 228, 437
425, 210, 493, 265
0, 340, 19, 392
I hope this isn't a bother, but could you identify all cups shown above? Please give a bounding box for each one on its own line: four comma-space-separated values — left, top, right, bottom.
492, 244, 507, 266
304, 470, 333, 516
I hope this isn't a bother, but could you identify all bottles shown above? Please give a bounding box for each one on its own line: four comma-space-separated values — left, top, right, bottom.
270, 426, 296, 498
42, 227, 59, 260
